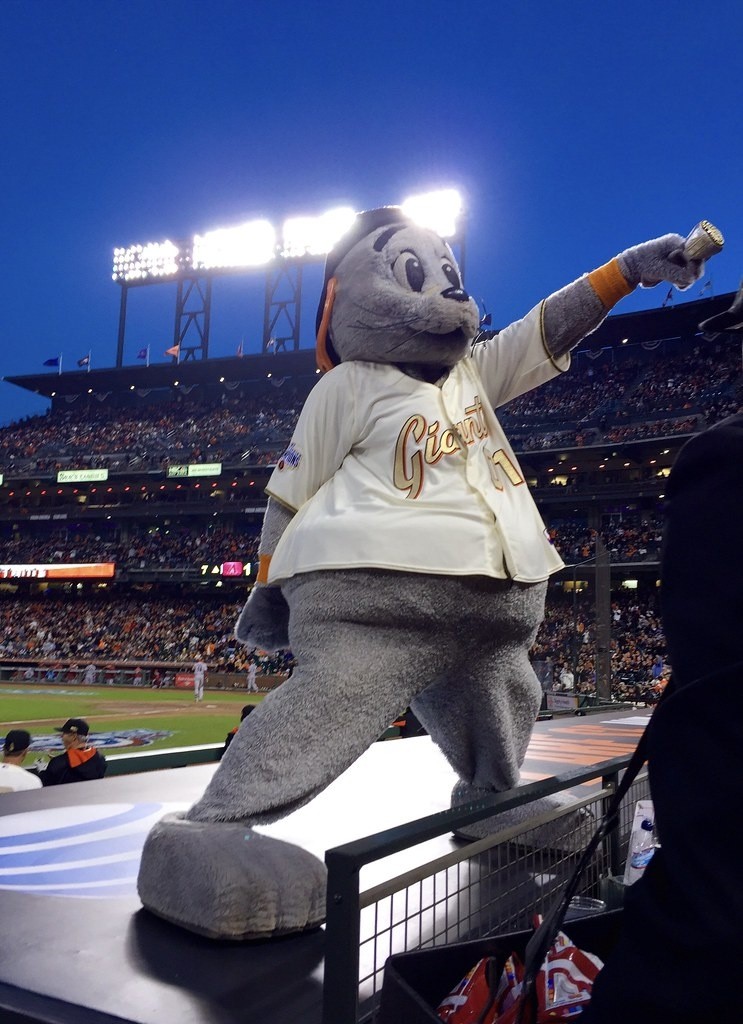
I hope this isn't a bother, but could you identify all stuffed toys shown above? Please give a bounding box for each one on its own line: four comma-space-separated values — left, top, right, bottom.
137, 208, 725, 939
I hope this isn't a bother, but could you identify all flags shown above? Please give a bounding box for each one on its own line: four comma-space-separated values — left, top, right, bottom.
266, 337, 274, 351
166, 345, 178, 358
237, 341, 243, 358
138, 348, 147, 360
42, 358, 59, 367
77, 356, 89, 368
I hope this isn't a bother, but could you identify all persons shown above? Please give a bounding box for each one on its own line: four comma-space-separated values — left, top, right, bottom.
637, 279, 743, 963
221, 705, 258, 759
192, 657, 207, 703
0, 337, 743, 704
36, 719, 107, 788
0, 729, 42, 797
247, 659, 259, 695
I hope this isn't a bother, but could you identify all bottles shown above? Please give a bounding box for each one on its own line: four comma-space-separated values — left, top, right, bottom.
627, 819, 653, 888
561, 896, 608, 922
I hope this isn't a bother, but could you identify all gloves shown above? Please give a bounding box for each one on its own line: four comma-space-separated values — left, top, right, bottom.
34, 759, 48, 773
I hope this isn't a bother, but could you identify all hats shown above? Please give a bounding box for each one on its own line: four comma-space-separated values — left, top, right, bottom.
3, 730, 35, 752
54, 718, 89, 736
